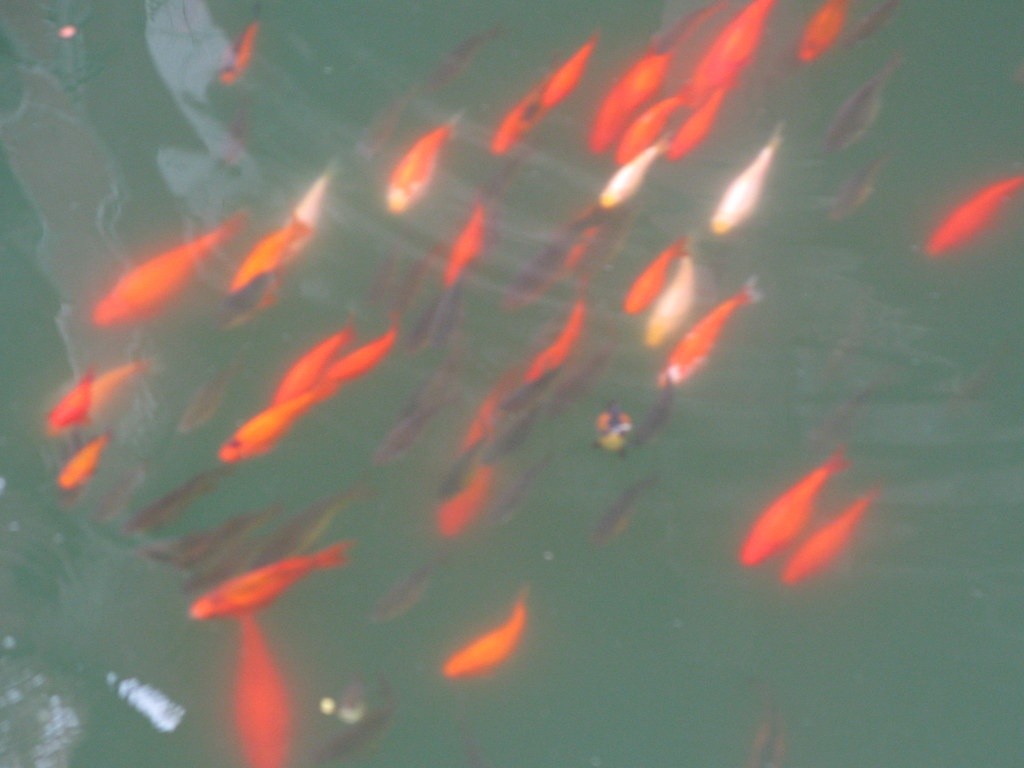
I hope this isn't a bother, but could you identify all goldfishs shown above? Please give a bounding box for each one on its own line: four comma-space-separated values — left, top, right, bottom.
42, 0, 1024, 768
